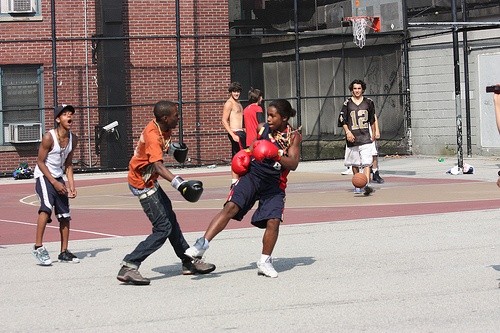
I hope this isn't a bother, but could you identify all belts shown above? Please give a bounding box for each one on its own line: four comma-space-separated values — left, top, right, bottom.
138, 183, 160, 201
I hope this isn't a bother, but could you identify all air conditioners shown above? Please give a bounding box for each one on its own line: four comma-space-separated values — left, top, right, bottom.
7, 0, 38, 14
8, 124, 43, 144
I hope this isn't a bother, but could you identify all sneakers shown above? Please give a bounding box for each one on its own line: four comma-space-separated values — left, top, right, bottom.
341, 166, 353, 174
30, 244, 52, 265
117, 266, 150, 285
182, 260, 216, 274
57, 249, 80, 263
373, 170, 384, 183
256, 255, 278, 278
184, 237, 206, 261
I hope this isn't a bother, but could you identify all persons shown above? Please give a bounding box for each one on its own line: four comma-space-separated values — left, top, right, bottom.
185, 99, 301, 278
244, 89, 266, 147
33, 104, 80, 265
222, 82, 247, 184
337, 80, 384, 196
116, 100, 216, 285
494, 84, 500, 176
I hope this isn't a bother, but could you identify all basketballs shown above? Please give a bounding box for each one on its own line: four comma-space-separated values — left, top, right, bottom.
352, 173, 367, 188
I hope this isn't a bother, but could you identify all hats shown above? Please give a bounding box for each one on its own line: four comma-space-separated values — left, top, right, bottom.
54, 104, 75, 118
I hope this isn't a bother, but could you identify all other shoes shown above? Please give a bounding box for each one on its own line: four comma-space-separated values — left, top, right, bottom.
354, 187, 376, 196
